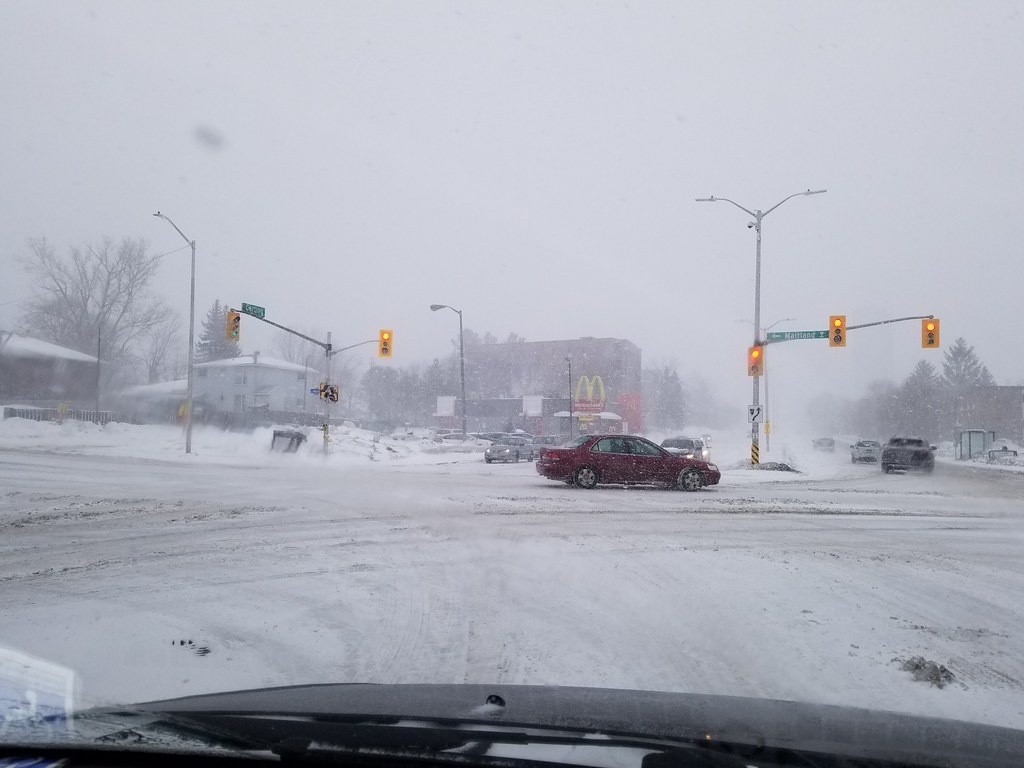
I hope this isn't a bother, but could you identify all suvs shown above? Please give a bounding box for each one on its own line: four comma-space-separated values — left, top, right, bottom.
425, 428, 533, 448
659, 435, 711, 461
531, 435, 563, 459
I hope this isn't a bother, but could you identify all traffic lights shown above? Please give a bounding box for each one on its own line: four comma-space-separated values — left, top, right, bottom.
319, 384, 329, 398
328, 387, 337, 401
226, 311, 241, 341
748, 346, 763, 376
922, 319, 940, 347
829, 315, 848, 347
379, 329, 393, 357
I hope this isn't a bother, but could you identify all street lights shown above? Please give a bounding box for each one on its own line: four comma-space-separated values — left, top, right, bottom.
737, 317, 797, 463
429, 303, 467, 440
153, 211, 198, 453
695, 187, 827, 465
565, 356, 574, 441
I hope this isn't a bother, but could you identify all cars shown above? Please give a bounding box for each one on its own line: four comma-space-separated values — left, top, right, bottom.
813, 436, 835, 452
881, 435, 938, 476
535, 435, 721, 492
484, 436, 534, 464
850, 439, 885, 464
